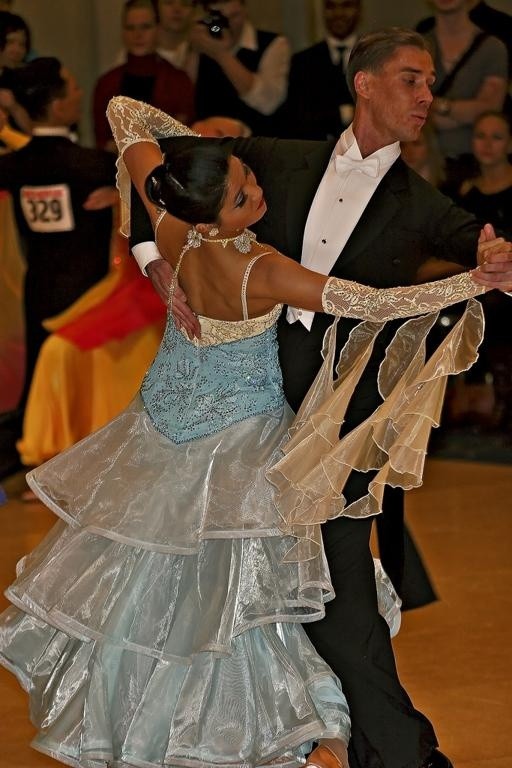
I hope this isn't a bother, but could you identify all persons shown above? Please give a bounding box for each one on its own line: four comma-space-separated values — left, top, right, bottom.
1, 90, 511, 767
0, 0, 360, 500
415, 0, 512, 464
126, 24, 512, 766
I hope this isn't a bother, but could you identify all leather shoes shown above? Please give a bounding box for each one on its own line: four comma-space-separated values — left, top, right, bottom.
305, 743, 344, 767
420, 746, 454, 767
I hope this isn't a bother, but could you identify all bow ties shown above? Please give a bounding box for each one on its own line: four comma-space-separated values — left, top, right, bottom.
334, 154, 380, 177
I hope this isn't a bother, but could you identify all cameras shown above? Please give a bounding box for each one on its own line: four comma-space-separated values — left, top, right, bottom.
200, 8, 230, 39
